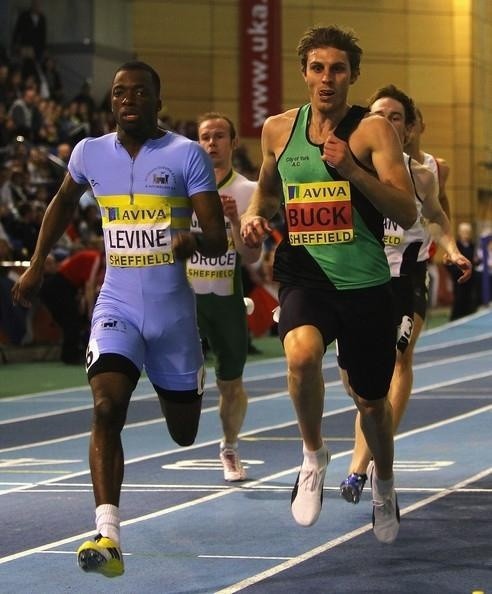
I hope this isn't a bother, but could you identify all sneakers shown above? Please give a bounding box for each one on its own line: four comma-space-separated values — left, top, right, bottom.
219, 440, 248, 482
77, 537, 125, 578
369, 464, 400, 545
291, 447, 331, 527
340, 473, 368, 504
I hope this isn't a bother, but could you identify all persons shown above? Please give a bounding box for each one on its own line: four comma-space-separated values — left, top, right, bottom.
340, 82, 476, 503
443, 221, 476, 320
188, 111, 260, 484
14, 59, 228, 577
239, 20, 419, 547
404, 103, 451, 261
